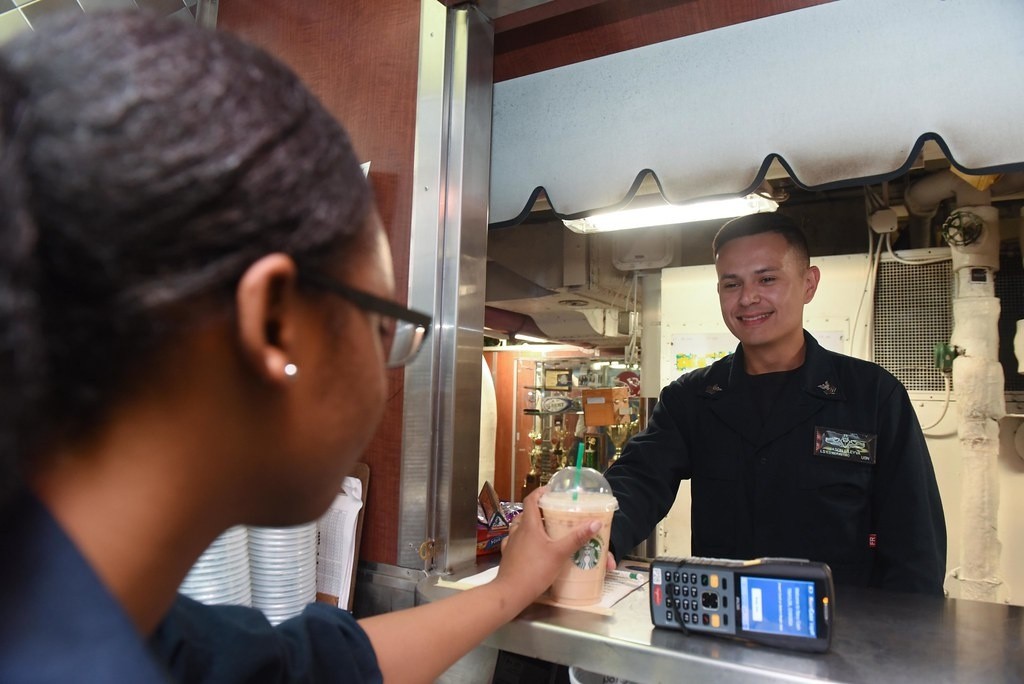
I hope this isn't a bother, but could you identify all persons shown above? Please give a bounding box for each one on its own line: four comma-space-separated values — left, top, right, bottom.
603, 213, 948, 598
0, 12, 602, 684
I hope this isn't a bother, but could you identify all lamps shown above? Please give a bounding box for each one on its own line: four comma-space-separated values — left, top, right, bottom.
561, 178, 789, 235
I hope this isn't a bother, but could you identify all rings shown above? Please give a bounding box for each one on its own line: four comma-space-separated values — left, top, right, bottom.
509, 521, 520, 529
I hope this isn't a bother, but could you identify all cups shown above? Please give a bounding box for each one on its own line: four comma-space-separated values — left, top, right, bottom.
569, 665, 634, 684
437, 645, 499, 684
539, 465, 618, 606
179, 524, 318, 625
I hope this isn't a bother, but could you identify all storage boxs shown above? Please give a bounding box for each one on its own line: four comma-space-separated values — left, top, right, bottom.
474, 524, 509, 556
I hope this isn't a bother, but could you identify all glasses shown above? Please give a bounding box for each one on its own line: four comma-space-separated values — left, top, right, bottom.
221, 264, 432, 369
